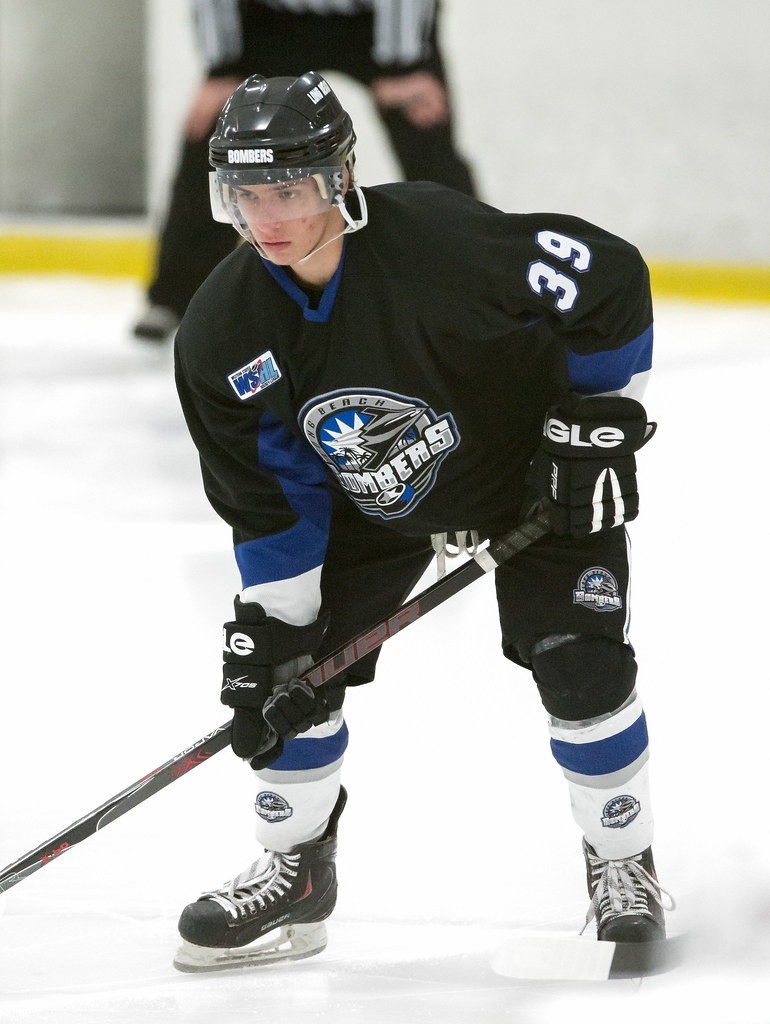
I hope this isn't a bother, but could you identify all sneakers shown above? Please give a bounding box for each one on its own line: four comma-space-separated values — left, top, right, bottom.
177, 784, 349, 952
566, 757, 669, 944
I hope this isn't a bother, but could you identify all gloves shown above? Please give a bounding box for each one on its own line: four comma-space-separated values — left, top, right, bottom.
221, 595, 333, 772
516, 395, 658, 541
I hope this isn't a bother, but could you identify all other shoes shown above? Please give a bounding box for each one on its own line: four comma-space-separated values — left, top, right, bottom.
132, 303, 179, 345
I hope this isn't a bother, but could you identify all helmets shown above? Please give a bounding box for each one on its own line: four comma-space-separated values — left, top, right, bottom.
207, 71, 358, 211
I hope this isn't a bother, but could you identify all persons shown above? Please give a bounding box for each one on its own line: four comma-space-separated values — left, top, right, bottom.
133, 0, 481, 340
173, 72, 665, 951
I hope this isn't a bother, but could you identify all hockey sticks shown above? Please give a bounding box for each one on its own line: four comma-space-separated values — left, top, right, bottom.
0, 420, 660, 895
